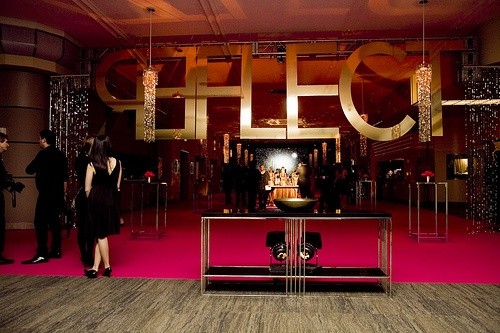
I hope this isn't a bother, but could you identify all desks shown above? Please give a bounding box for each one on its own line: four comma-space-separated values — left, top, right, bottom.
408, 182, 449, 243
129, 182, 167, 240
356, 180, 376, 206
201, 209, 392, 298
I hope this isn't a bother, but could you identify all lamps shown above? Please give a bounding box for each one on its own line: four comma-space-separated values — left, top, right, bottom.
266, 231, 291, 273
359, 81, 369, 157
416, 0, 432, 142
224, 133, 230, 163
298, 232, 322, 271
143, 8, 158, 144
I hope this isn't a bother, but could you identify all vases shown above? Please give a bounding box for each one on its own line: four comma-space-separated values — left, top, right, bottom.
148, 177, 150, 183
426, 176, 430, 182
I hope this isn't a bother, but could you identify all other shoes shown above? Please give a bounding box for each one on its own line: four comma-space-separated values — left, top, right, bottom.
0, 254, 14, 263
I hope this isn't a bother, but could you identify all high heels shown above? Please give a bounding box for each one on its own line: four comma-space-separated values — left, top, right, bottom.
103, 267, 111, 277
84, 268, 96, 278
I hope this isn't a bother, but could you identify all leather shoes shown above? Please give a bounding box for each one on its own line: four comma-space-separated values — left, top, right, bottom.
49, 251, 60, 257
21, 255, 48, 264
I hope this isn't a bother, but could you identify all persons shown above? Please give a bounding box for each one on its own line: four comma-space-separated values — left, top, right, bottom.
75, 136, 95, 266
0, 132, 15, 264
84, 134, 122, 277
296, 157, 313, 198
224, 157, 262, 209
317, 163, 370, 213
259, 164, 270, 208
21, 130, 69, 264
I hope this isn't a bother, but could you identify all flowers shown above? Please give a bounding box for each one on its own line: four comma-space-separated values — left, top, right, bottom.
421, 171, 434, 178
144, 171, 155, 178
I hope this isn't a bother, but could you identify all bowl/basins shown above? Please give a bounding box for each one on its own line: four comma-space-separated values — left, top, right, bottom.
273, 197, 318, 213
